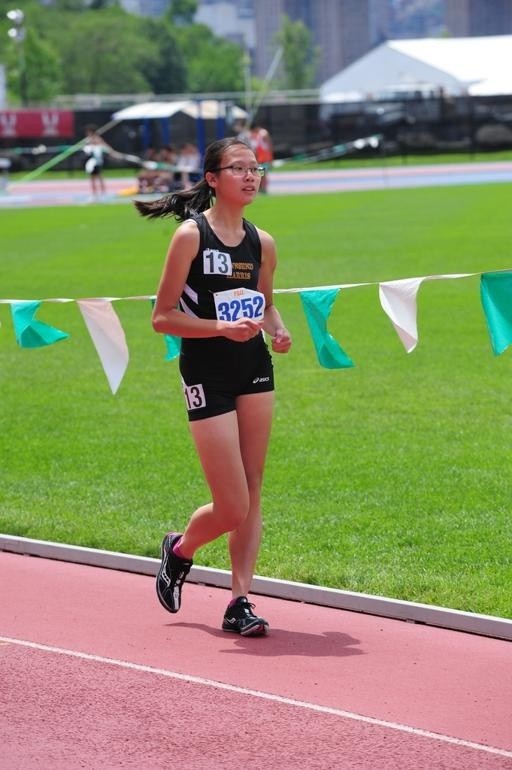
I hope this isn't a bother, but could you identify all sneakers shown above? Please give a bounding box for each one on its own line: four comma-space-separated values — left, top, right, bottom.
222, 595, 269, 637
156, 532, 193, 612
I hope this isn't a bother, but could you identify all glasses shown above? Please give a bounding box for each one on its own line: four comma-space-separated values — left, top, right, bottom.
212, 162, 265, 177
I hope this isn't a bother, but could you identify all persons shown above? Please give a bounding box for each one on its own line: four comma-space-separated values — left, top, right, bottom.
79, 116, 275, 198
131, 137, 292, 639
